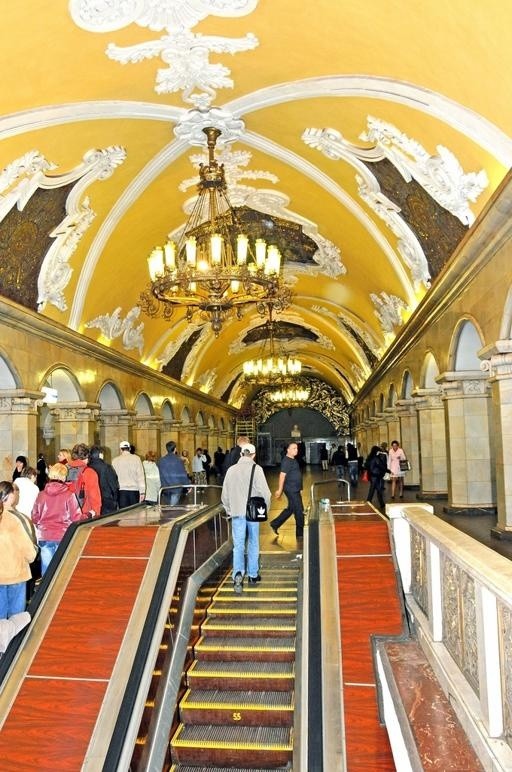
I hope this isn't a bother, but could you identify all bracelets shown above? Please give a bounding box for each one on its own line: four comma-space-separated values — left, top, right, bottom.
140, 493, 144, 495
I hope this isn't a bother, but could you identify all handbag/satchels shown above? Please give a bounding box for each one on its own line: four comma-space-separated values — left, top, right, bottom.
362, 470, 368, 482
399, 459, 411, 471
383, 472, 391, 481
246, 496, 268, 522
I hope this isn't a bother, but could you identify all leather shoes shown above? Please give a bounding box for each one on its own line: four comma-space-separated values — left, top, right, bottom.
248, 575, 262, 584
269, 522, 279, 535
233, 572, 243, 594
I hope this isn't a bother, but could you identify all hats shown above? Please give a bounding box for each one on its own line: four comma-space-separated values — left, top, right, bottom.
242, 444, 256, 454
120, 441, 130, 448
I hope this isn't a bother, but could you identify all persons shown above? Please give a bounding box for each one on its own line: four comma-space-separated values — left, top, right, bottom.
191, 447, 208, 496
13, 466, 41, 601
31, 463, 96, 579
12, 456, 27, 482
268, 441, 304, 540
230, 437, 250, 541
87, 445, 119, 517
272, 436, 408, 559
0, 480, 39, 622
58, 449, 71, 466
202, 449, 211, 485
64, 442, 102, 517
214, 446, 234, 486
221, 443, 271, 594
174, 450, 189, 493
182, 449, 193, 480
143, 451, 161, 506
36, 453, 47, 491
156, 441, 191, 506
112, 441, 145, 509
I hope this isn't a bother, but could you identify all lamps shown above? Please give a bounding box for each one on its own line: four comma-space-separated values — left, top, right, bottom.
237, 292, 310, 386
264, 382, 314, 416
136, 122, 295, 339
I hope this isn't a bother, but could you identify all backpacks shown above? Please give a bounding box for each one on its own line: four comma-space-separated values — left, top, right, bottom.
65, 464, 87, 508
